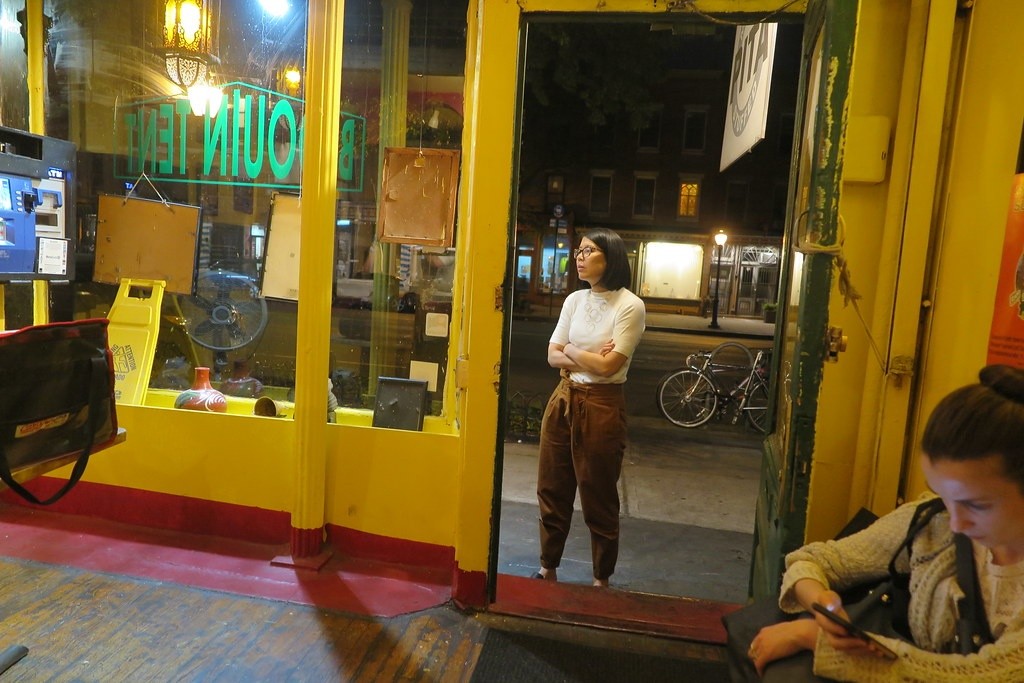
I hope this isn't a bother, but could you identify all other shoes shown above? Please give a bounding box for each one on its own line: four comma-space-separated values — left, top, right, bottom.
530, 572, 544, 580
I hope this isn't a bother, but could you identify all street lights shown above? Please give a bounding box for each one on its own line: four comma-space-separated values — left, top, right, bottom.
707, 227, 727, 329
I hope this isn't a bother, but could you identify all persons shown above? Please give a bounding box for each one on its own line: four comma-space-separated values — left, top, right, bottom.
530, 226, 647, 588
748, 362, 1024, 682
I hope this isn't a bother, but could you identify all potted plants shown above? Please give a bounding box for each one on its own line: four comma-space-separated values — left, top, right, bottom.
764, 302, 777, 324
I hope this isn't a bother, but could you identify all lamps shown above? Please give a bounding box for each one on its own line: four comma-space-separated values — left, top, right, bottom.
149, 0, 221, 91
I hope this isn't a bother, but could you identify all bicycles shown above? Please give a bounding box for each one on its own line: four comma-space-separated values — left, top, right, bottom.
655, 348, 773, 435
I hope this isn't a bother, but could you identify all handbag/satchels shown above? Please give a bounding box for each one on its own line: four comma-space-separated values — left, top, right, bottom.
0, 318, 119, 506
719, 488, 948, 683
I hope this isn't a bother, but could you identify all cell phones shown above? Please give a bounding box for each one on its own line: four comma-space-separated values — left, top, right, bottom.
810, 603, 897, 662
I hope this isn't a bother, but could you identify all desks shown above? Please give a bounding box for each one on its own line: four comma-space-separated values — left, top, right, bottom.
0, 425, 127, 678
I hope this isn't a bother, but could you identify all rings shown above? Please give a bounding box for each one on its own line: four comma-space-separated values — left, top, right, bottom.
752, 651, 757, 659
750, 644, 753, 650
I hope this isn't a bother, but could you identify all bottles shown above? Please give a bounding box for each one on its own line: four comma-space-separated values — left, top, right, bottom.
175, 368, 227, 412
220, 358, 262, 399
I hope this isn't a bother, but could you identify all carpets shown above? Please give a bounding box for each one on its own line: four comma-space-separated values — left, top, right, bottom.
466, 626, 734, 683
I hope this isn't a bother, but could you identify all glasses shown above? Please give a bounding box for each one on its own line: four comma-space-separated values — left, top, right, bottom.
573, 247, 604, 259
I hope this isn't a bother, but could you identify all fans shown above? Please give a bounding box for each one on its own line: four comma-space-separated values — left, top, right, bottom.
178, 270, 267, 375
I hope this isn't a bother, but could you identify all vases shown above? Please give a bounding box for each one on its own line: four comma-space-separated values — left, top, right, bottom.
174, 367, 227, 413
219, 357, 264, 399
